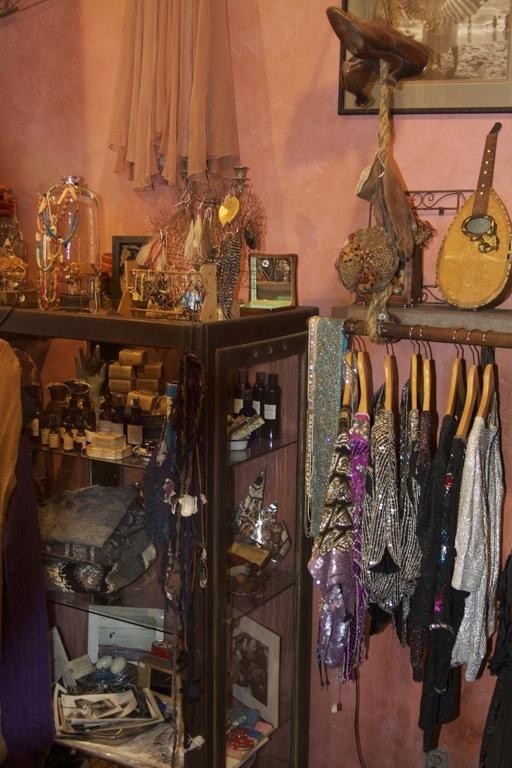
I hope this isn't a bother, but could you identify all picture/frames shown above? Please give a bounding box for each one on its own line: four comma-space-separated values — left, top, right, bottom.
337, 0, 512, 115
111, 235, 153, 307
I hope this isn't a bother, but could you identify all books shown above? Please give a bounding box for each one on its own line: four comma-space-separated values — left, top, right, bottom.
225, 709, 272, 761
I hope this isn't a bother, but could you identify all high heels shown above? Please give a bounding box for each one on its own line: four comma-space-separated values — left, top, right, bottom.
326, 6, 429, 88
339, 57, 371, 106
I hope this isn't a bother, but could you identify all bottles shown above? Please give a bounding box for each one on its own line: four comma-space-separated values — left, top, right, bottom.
97, 393, 145, 446
232, 370, 284, 440
35, 174, 101, 317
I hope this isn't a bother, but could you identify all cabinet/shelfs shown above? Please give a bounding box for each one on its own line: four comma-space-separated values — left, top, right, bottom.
0, 302, 319, 768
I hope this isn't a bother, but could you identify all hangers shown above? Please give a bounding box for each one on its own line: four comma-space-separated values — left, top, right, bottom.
342, 318, 498, 440
